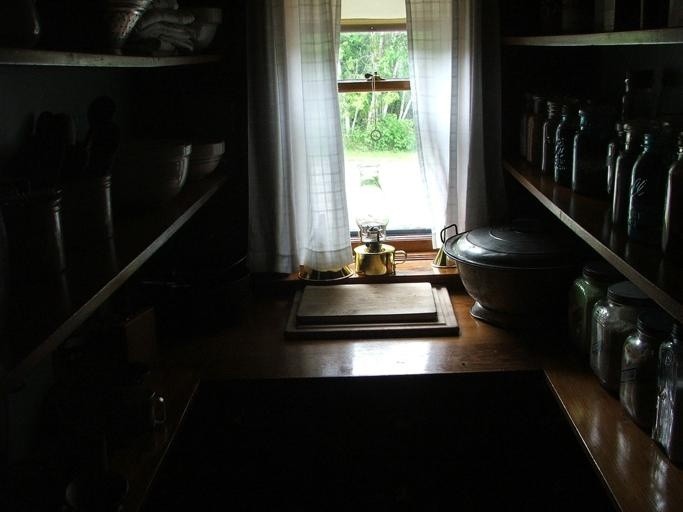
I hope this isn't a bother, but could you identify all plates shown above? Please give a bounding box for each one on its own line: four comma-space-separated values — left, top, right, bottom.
64, 468, 130, 511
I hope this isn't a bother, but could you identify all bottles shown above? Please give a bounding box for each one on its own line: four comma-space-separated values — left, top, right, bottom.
568, 257, 683, 464
519, 68, 683, 257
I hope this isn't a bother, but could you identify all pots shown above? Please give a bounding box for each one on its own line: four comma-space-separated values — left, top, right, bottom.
440, 219, 592, 329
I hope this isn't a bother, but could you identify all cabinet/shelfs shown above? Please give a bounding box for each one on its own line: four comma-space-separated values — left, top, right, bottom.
0, 47, 226, 397
503, 26, 683, 326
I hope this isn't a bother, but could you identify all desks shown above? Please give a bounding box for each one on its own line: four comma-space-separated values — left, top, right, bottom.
0, 260, 683, 512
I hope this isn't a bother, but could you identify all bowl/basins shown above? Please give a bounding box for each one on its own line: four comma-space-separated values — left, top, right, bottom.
169, 134, 225, 186
93, 136, 192, 208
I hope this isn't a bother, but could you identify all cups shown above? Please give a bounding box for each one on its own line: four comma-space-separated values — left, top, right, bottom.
121, 386, 166, 434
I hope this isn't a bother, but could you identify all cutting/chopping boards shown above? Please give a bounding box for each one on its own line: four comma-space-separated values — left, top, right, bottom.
296, 282, 438, 322
284, 281, 459, 340
